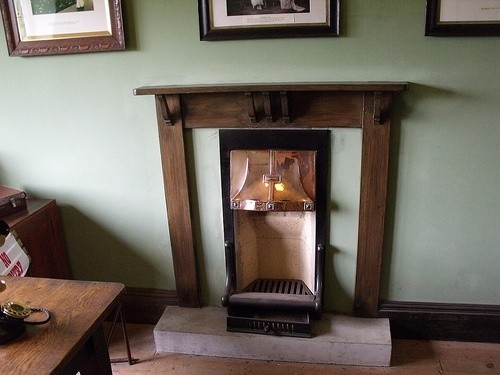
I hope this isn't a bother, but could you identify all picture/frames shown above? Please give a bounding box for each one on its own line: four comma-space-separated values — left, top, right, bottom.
196, 0, 341, 41
423, 0, 500, 40
0, 0, 128, 58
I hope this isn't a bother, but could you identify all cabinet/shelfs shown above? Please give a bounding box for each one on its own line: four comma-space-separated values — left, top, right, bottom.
0, 185, 75, 280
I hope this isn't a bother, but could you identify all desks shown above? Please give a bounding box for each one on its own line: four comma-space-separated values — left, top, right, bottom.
0, 274, 129, 375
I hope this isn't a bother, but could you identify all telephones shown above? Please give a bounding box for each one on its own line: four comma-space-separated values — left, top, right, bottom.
0, 279, 50, 345
